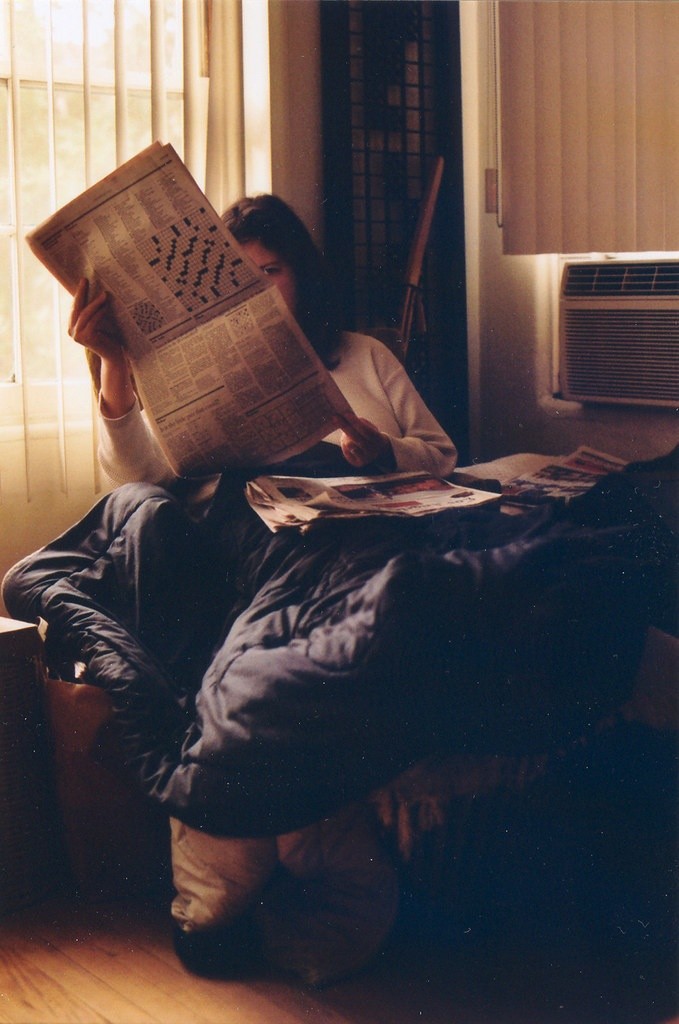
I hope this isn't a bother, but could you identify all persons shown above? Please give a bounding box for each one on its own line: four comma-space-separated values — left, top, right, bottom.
71, 196, 458, 484
